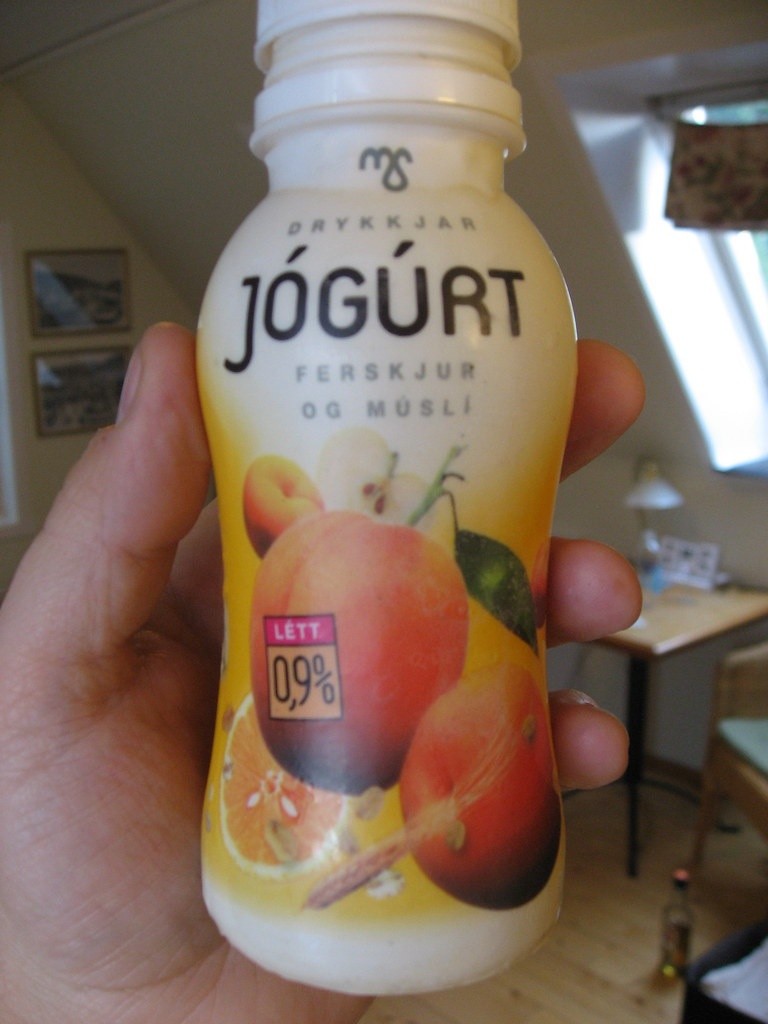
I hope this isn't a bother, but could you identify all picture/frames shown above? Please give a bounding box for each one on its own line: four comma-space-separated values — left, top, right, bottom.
22, 248, 134, 338
30, 345, 134, 438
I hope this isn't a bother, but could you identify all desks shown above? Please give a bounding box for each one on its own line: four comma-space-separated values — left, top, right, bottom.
582, 582, 767, 876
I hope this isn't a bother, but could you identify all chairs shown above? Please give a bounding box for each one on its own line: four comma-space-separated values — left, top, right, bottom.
685, 642, 768, 880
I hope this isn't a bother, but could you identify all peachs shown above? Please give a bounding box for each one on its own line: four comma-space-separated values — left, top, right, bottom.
243, 456, 561, 909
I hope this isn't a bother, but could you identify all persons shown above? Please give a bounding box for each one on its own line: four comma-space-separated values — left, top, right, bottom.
1, 320, 647, 1023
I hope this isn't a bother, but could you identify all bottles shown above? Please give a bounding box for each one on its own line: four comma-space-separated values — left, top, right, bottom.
195, 1, 578, 994
636, 529, 666, 625
658, 868, 695, 977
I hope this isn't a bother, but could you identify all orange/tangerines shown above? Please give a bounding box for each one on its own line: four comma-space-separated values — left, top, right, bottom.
219, 692, 353, 877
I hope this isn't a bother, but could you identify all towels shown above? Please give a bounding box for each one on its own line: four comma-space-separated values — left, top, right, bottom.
664, 120, 768, 232
